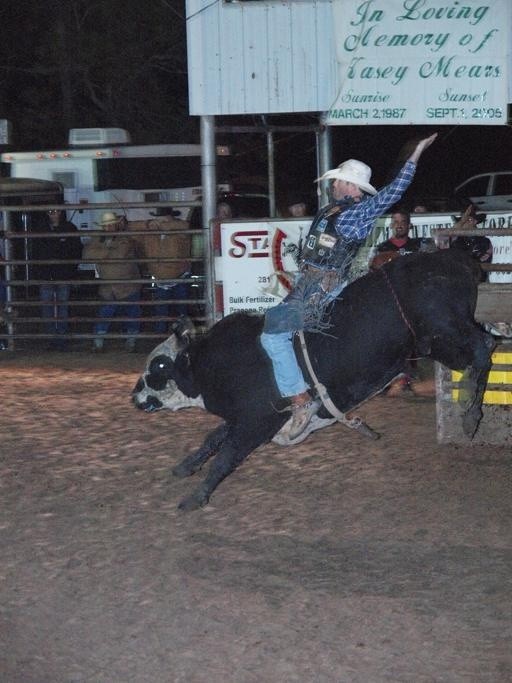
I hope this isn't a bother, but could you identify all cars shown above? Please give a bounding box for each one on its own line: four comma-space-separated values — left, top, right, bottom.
453, 169, 511, 213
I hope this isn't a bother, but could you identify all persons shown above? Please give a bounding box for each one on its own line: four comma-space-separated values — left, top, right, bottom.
30, 209, 84, 352
449, 197, 493, 283
129, 207, 193, 334
287, 196, 306, 217
80, 210, 144, 353
259, 131, 438, 440
370, 211, 423, 398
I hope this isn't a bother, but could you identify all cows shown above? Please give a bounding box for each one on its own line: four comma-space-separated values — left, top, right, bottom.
128, 238, 505, 516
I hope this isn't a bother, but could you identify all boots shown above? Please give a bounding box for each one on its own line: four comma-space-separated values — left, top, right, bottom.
92, 331, 107, 353
123, 330, 138, 354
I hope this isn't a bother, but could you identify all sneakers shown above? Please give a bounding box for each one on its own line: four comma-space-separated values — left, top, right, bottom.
288, 395, 322, 441
45, 338, 67, 351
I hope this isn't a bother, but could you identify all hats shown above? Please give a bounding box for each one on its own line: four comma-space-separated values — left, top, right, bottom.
97, 212, 121, 227
314, 156, 378, 196
451, 205, 487, 224
149, 206, 181, 218
